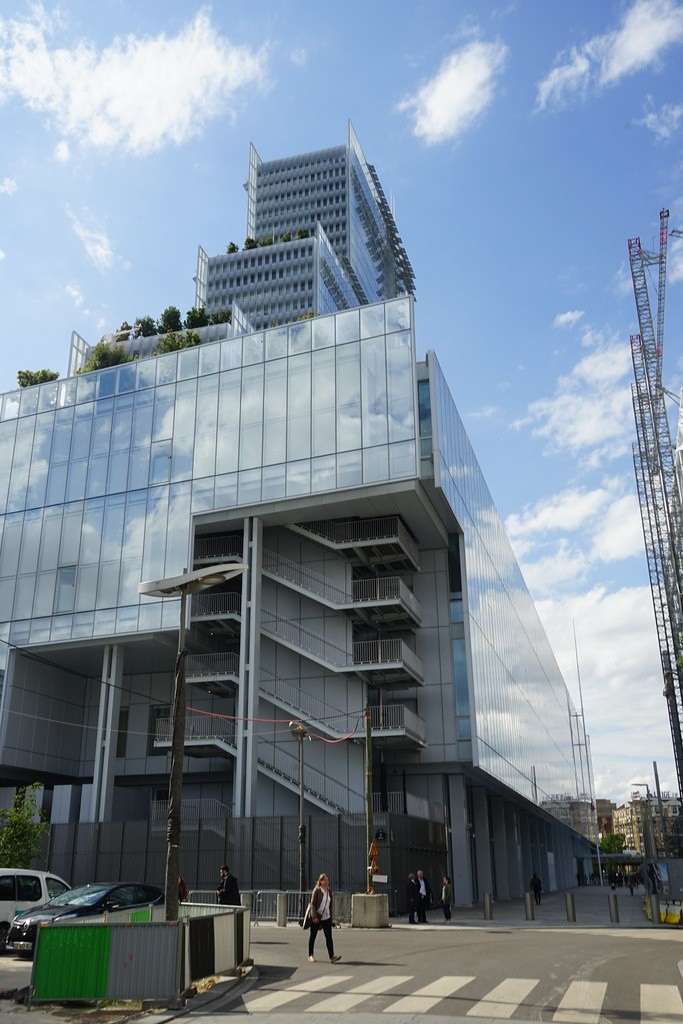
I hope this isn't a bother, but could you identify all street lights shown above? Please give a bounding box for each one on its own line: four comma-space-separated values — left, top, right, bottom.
288, 721, 309, 926
632, 784, 657, 860
137, 563, 249, 921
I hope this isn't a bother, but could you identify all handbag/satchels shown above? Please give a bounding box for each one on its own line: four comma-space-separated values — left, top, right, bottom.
306, 910, 322, 928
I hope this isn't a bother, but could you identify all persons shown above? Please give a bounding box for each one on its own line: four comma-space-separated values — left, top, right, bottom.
216, 865, 241, 906
441, 876, 452, 924
415, 870, 432, 923
178, 876, 186, 902
530, 872, 542, 906
577, 872, 600, 886
407, 873, 419, 924
614, 872, 641, 896
307, 873, 341, 963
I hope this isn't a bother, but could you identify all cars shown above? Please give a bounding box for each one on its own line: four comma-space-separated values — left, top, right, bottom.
7, 882, 165, 958
0, 868, 83, 957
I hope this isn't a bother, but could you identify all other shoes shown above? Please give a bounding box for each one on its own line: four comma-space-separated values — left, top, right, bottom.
331, 955, 341, 963
444, 919, 452, 924
308, 956, 316, 962
409, 921, 418, 924
418, 919, 429, 923
